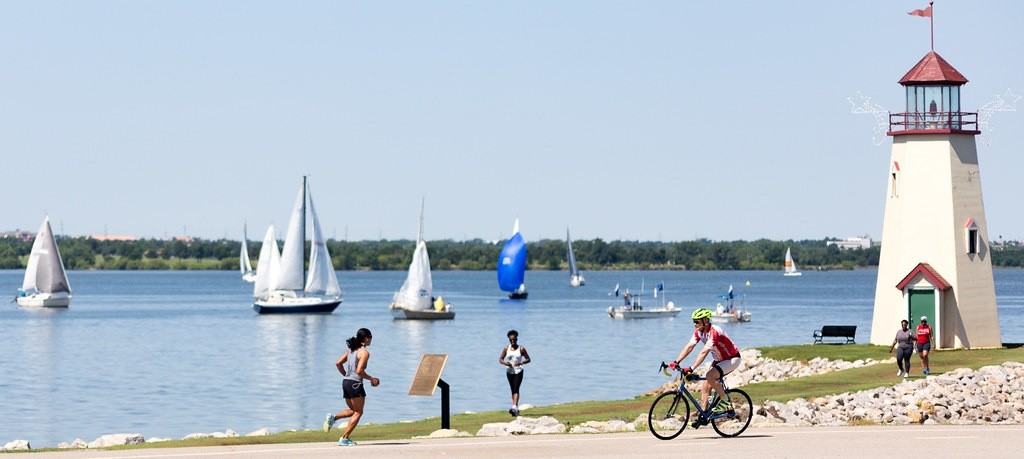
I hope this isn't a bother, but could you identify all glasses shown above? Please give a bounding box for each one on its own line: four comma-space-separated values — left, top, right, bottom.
901, 323, 907, 327
693, 320, 701, 324
920, 320, 927, 323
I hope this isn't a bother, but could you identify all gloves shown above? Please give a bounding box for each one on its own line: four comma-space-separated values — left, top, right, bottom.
669, 362, 680, 370
683, 367, 693, 376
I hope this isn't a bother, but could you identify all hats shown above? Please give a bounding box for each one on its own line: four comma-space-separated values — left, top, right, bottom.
920, 316, 927, 321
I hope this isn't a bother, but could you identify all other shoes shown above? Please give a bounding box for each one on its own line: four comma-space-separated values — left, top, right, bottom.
509, 407, 520, 417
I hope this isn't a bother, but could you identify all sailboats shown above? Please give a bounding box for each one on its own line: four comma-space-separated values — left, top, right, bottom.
389, 193, 456, 321
16, 214, 72, 308
567, 227, 585, 287
240, 222, 256, 282
497, 233, 529, 299
253, 175, 344, 313
783, 246, 802, 276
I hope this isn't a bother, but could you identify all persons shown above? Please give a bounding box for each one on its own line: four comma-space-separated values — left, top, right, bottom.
324, 328, 379, 447
668, 309, 741, 427
914, 316, 936, 375
717, 303, 725, 314
888, 320, 917, 378
499, 330, 530, 416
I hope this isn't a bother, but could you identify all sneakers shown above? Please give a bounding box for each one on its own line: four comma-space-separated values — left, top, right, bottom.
896, 368, 904, 377
324, 414, 335, 434
687, 409, 706, 421
904, 372, 909, 378
710, 401, 732, 412
919, 367, 929, 374
338, 436, 357, 446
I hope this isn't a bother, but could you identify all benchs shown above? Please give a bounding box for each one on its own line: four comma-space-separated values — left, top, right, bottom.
813, 325, 857, 344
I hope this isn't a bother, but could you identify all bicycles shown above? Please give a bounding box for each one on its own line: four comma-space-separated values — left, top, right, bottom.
648, 361, 753, 438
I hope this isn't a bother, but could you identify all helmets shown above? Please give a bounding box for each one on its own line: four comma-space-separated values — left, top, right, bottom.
691, 307, 712, 320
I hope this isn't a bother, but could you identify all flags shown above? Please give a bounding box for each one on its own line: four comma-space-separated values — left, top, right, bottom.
729, 290, 733, 299
615, 285, 619, 296
658, 284, 663, 291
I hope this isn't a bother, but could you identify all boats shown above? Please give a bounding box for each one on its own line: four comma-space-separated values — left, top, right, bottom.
710, 285, 752, 323
608, 277, 682, 319
